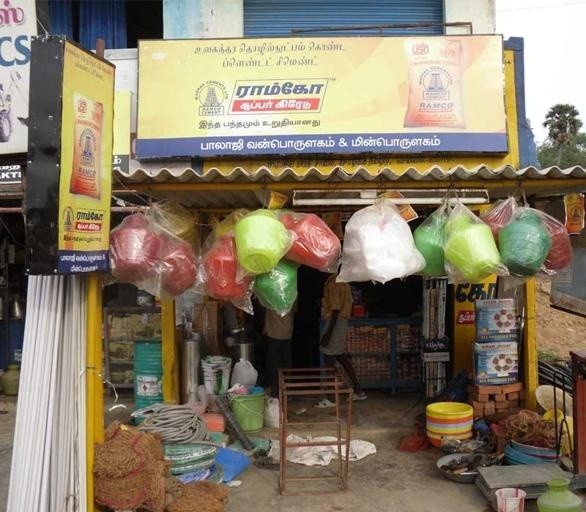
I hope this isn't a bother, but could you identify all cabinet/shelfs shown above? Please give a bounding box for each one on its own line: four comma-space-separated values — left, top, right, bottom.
320, 318, 423, 395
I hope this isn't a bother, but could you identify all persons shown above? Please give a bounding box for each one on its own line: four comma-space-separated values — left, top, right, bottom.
313, 272, 367, 409
263, 301, 298, 403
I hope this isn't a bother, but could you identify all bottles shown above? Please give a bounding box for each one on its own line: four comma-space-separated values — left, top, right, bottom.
535, 477, 583, 511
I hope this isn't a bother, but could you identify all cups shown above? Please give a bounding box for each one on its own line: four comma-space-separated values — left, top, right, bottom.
495, 488, 525, 512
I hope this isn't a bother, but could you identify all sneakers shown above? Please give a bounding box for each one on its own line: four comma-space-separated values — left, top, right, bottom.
313, 396, 338, 408
347, 391, 368, 401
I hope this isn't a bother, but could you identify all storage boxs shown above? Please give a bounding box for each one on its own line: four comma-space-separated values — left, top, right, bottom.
471, 341, 518, 385
475, 298, 519, 342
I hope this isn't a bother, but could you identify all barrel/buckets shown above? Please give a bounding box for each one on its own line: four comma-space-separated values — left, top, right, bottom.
132, 340, 164, 425
230, 358, 258, 389
229, 391, 265, 433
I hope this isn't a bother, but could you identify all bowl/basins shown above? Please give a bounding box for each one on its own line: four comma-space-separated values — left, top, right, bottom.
504, 439, 560, 467
425, 401, 474, 449
